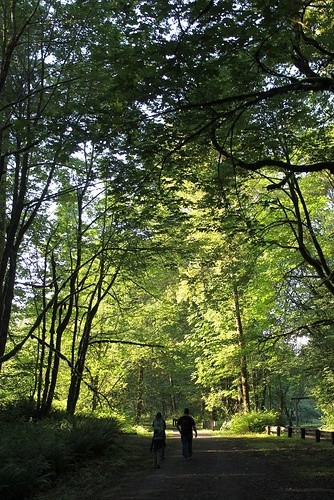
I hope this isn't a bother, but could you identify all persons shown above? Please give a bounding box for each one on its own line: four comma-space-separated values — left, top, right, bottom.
175, 408, 198, 460
151, 412, 166, 469
